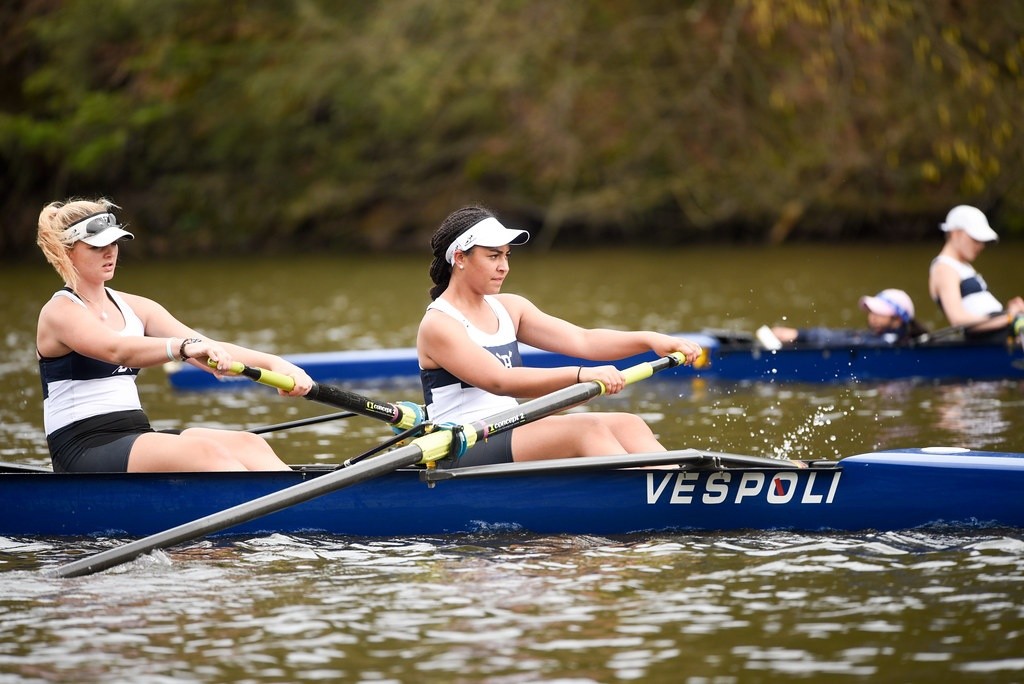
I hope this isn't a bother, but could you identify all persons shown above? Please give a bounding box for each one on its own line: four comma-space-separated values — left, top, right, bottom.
417, 204, 704, 469
36, 197, 312, 472
773, 287, 927, 348
927, 205, 1024, 344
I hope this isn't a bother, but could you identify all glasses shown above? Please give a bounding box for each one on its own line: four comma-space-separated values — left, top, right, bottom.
79, 213, 116, 240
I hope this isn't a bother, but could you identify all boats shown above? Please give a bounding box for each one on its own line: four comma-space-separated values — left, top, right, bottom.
0, 448, 1024, 543
163, 332, 1024, 390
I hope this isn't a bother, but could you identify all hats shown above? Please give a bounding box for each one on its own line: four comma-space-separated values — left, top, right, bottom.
445, 217, 530, 267
941, 206, 998, 242
56, 211, 134, 247
860, 289, 915, 323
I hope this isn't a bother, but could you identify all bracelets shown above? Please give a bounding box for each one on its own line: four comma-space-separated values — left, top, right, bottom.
166, 337, 176, 361
577, 365, 584, 383
180, 339, 202, 361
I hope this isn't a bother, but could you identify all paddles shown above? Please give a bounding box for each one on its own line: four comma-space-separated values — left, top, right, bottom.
205, 355, 428, 436
41, 351, 686, 582
